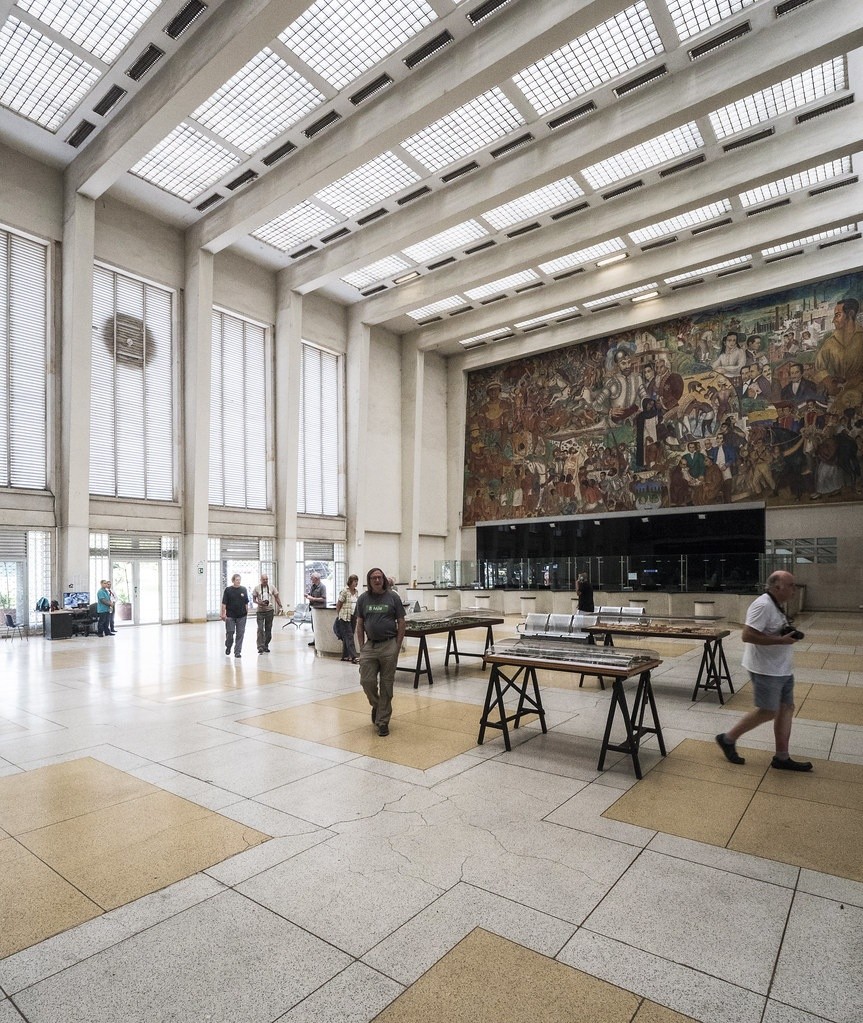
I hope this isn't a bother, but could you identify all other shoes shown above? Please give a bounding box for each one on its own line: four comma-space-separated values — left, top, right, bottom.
225, 648, 231, 655
97, 633, 103, 637
105, 632, 115, 636
264, 648, 270, 652
308, 642, 315, 646
235, 653, 241, 658
341, 657, 351, 661
258, 649, 264, 653
111, 629, 117, 632
352, 658, 360, 664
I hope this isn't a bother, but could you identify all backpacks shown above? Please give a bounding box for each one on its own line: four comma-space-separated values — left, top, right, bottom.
35, 597, 49, 612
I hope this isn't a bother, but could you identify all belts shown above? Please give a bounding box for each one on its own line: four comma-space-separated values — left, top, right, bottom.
367, 635, 397, 644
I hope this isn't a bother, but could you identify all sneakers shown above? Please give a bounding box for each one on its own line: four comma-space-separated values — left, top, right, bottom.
715, 734, 745, 764
371, 708, 377, 724
771, 756, 813, 771
377, 725, 389, 736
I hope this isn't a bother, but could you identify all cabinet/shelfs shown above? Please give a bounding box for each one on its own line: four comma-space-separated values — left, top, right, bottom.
42, 607, 100, 640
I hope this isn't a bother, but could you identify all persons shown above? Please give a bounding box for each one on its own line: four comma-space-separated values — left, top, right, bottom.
353, 568, 407, 736
253, 575, 282, 653
337, 574, 398, 663
304, 572, 326, 645
96, 580, 117, 637
220, 574, 250, 658
715, 571, 813, 770
576, 572, 594, 614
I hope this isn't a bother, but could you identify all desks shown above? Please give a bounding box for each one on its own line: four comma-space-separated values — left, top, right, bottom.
477, 648, 666, 780
579, 625, 735, 705
393, 617, 505, 689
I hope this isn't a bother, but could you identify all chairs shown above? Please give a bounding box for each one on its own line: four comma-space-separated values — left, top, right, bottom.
513, 606, 652, 645
73, 602, 99, 637
5, 614, 29, 644
281, 602, 314, 633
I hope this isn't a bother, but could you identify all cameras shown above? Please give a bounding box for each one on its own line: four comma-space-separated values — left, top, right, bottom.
264, 600, 269, 606
780, 627, 804, 640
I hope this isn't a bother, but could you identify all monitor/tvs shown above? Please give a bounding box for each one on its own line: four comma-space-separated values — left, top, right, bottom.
64, 592, 90, 610
